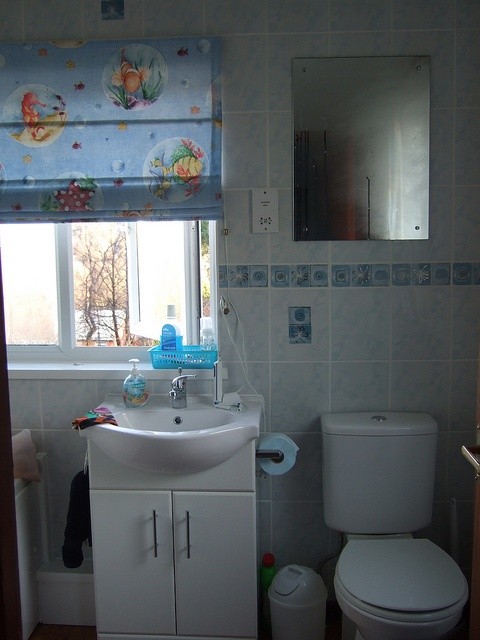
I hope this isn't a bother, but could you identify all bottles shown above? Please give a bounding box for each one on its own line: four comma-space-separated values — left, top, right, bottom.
198, 316, 215, 352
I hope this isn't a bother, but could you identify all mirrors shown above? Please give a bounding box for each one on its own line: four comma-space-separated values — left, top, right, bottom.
290, 56, 430, 242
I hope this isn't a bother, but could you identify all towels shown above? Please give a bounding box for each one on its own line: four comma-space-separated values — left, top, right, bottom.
62, 470, 92, 569
72, 407, 119, 432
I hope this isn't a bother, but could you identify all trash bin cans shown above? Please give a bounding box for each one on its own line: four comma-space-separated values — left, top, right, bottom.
267, 564, 329, 640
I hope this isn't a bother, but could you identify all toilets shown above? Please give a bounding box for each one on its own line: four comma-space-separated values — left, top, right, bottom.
320, 412, 470, 638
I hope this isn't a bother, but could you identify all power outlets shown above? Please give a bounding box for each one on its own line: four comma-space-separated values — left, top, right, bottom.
251, 187, 279, 233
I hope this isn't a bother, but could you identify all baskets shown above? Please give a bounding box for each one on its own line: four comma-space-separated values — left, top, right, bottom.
147, 335, 217, 369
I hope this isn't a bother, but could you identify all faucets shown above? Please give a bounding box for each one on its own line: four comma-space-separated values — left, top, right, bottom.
168, 374, 197, 408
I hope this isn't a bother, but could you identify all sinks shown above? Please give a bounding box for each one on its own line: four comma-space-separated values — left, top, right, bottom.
80, 397, 260, 472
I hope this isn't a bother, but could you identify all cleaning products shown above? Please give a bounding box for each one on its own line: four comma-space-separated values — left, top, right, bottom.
199, 316, 215, 350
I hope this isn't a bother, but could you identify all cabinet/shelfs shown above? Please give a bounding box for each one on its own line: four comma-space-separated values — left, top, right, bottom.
89, 490, 258, 640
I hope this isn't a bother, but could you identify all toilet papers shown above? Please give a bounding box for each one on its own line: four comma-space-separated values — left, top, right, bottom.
259, 431, 299, 476
